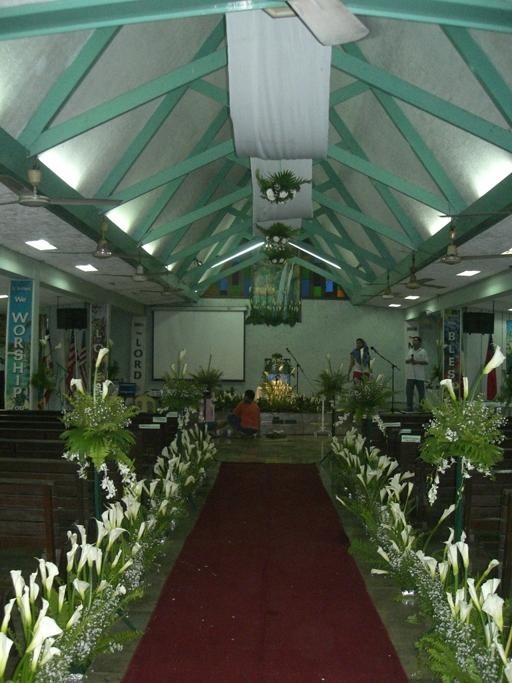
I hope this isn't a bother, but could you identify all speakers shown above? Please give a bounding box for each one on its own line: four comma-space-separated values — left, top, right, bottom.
56, 308, 87, 329
463, 312, 494, 335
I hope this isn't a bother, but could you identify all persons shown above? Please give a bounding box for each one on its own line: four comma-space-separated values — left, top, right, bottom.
197, 387, 216, 430
347, 338, 373, 384
227, 390, 261, 438
403, 337, 429, 412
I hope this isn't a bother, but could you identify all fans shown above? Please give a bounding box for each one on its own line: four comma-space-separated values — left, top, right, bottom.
1, 169, 185, 296
357, 211, 512, 299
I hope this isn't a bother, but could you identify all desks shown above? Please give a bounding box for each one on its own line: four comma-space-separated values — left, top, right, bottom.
215, 407, 334, 435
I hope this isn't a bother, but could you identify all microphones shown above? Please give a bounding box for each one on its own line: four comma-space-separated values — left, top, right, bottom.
369, 345, 379, 355
285, 346, 295, 359
411, 352, 415, 364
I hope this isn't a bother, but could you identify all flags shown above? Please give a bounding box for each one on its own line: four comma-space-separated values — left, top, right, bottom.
43, 329, 54, 400
485, 334, 497, 401
65, 330, 76, 395
79, 333, 88, 389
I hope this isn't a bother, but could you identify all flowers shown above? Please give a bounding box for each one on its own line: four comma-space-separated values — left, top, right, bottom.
2, 420, 220, 680
148, 347, 209, 419
256, 168, 312, 207
263, 222, 301, 265
329, 426, 509, 682
344, 346, 402, 427
313, 352, 351, 403
415, 347, 510, 506
57, 347, 138, 498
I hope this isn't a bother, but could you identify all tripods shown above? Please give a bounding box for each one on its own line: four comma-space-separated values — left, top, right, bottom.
374, 356, 406, 415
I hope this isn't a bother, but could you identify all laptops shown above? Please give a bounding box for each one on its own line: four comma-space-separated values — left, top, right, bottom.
118, 382, 136, 397
